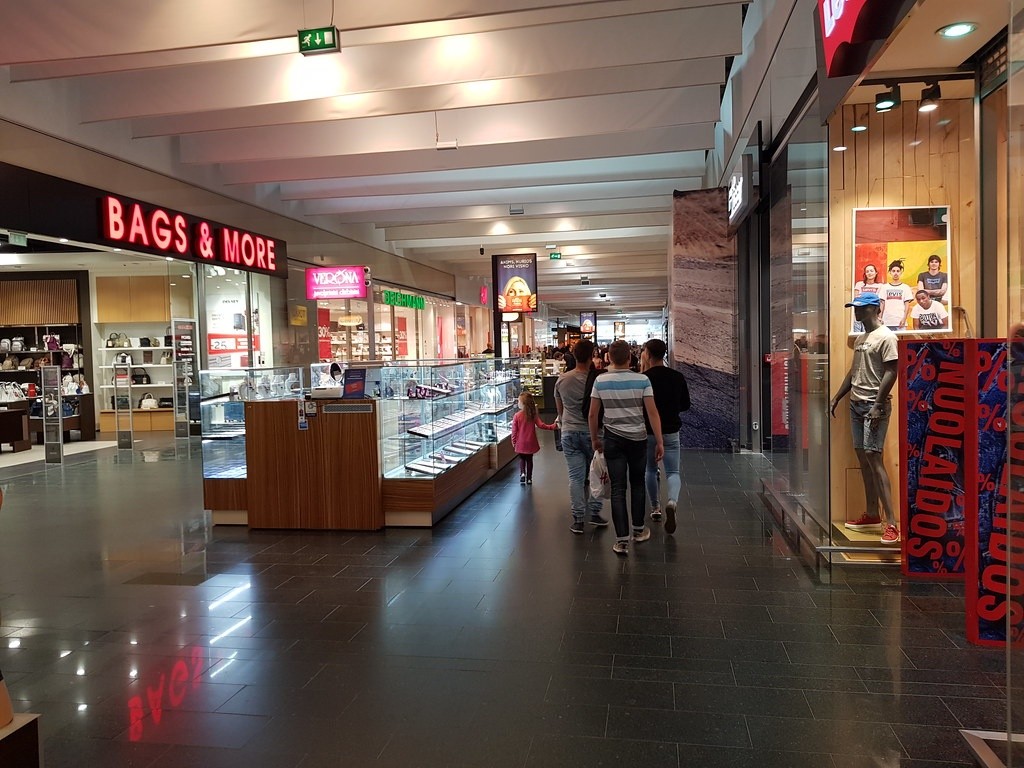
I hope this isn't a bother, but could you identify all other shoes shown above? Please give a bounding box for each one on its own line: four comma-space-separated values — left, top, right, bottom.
520, 477, 525, 485
587, 516, 609, 526
569, 522, 583, 533
632, 525, 651, 541
665, 505, 676, 535
650, 510, 662, 523
527, 479, 532, 484
612, 542, 629, 553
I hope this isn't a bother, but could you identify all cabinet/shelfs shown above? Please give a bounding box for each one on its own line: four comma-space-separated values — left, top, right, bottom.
0, 270, 566, 530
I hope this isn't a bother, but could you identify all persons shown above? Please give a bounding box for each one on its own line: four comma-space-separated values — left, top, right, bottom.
512, 391, 558, 485
482, 342, 494, 354
825, 293, 899, 544
498, 275, 536, 312
589, 340, 664, 555
854, 254, 948, 330
794, 334, 829, 354
641, 339, 692, 534
554, 339, 610, 534
513, 340, 645, 372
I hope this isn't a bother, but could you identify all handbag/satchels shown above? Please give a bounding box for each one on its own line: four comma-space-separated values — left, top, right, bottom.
106, 326, 173, 409
42, 332, 78, 358
587, 450, 611, 502
0, 339, 11, 351
0, 352, 89, 417
10, 335, 24, 352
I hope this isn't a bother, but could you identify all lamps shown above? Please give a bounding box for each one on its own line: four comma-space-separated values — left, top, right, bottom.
875, 84, 901, 113
599, 293, 606, 297
919, 81, 941, 111
617, 309, 622, 313
581, 275, 590, 285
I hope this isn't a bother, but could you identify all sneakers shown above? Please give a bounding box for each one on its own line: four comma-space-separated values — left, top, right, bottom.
845, 512, 881, 528
880, 524, 899, 544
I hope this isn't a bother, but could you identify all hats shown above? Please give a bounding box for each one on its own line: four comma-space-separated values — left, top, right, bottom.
845, 293, 880, 307
